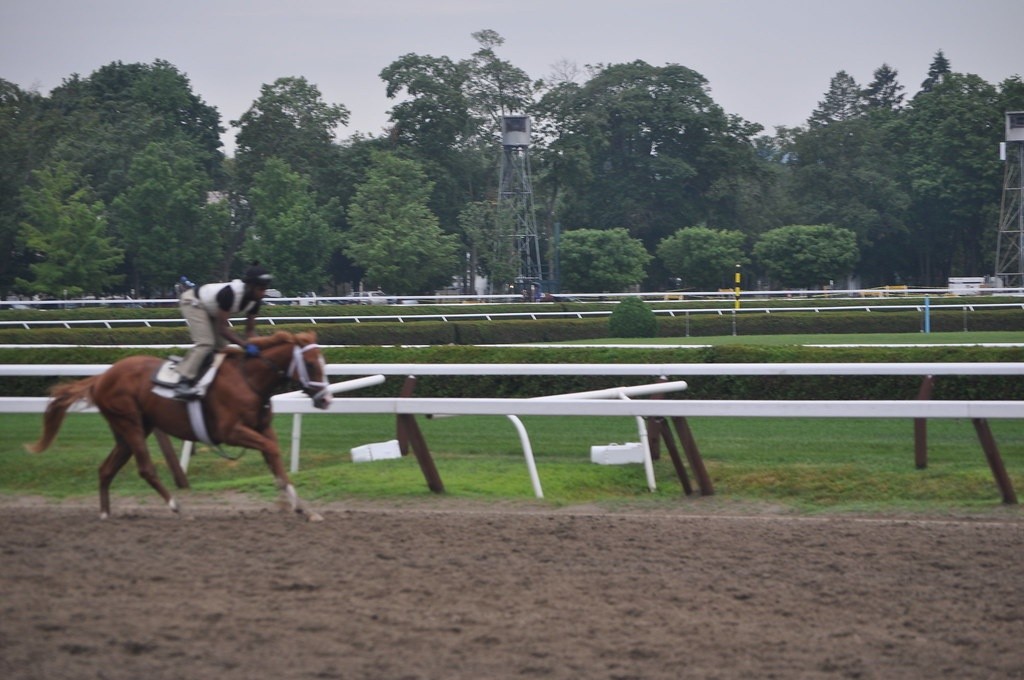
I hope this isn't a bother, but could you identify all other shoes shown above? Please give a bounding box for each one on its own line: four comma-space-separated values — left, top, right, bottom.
175, 381, 200, 395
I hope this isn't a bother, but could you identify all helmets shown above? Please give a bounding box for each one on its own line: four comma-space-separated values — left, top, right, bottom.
243, 266, 272, 282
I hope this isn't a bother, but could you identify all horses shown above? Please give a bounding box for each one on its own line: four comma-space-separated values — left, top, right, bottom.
21, 329, 334, 523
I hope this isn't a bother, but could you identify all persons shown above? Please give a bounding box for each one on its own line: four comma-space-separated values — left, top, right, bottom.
173, 266, 274, 395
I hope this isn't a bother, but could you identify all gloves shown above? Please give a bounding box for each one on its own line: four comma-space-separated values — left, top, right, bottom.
246, 344, 260, 357
